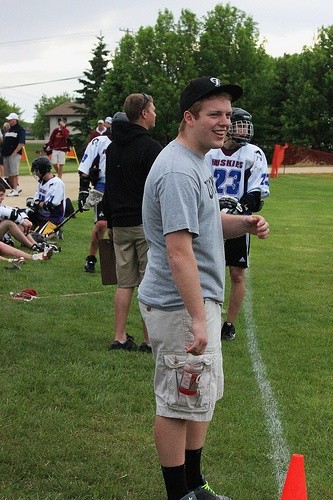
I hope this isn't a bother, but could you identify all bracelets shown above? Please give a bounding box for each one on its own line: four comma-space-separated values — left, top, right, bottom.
8, 258, 13, 263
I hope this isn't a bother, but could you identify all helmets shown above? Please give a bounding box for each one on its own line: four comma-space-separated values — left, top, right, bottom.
112, 112, 131, 122
226, 108, 254, 144
30, 156, 52, 184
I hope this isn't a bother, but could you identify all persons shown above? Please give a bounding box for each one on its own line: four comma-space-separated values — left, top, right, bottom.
16, 156, 66, 225
205, 108, 271, 340
0, 184, 53, 263
77, 128, 113, 273
46, 117, 69, 178
137, 77, 270, 500
89, 117, 112, 140
103, 93, 164, 353
0, 112, 25, 197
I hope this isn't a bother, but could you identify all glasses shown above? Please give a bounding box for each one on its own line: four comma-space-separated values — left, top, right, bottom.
140, 93, 150, 116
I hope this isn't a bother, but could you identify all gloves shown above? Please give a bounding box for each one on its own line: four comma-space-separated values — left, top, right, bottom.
78, 188, 91, 213
26, 201, 40, 207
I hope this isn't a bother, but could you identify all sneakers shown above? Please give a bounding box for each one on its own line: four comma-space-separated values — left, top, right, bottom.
220, 322, 236, 342
7, 189, 19, 197
110, 339, 152, 353
17, 186, 22, 193
179, 474, 231, 500
85, 255, 98, 273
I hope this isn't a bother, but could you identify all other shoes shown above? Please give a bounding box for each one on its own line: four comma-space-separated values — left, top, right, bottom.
33, 247, 53, 261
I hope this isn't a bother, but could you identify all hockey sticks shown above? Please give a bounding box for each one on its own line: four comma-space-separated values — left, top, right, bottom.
44, 189, 104, 237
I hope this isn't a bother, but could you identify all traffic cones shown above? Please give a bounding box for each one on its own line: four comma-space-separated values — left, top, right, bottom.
279, 452, 309, 500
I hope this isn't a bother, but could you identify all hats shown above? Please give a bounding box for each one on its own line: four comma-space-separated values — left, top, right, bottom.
98, 120, 104, 123
180, 76, 244, 120
5, 113, 18, 120
105, 117, 112, 123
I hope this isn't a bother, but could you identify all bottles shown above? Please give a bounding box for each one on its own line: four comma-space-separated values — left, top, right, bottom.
177, 343, 204, 403
